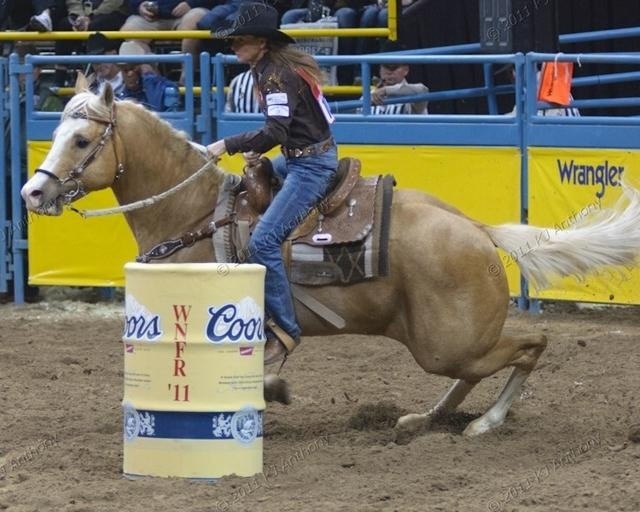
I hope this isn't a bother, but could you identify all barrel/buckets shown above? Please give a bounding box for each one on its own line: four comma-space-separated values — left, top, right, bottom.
121, 262, 268, 483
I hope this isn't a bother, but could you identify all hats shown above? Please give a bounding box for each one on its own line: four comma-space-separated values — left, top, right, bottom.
378, 40, 410, 69
85, 32, 114, 55
225, 2, 296, 44
9, 42, 40, 63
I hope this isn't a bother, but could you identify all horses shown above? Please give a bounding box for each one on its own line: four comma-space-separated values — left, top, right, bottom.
19, 72, 640, 440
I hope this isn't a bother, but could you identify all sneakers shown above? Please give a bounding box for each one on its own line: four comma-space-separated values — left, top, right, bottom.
55, 63, 85, 75
30, 14, 53, 31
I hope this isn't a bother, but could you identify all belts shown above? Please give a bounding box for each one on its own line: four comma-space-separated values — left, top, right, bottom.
280, 135, 333, 160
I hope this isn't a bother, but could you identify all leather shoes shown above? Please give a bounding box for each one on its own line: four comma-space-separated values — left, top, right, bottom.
264, 333, 301, 364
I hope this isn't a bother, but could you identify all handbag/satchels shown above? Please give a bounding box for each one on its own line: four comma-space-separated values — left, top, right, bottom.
308, 0, 323, 23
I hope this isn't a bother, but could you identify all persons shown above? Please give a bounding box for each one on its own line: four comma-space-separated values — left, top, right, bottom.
206, 0, 338, 365
506, 60, 584, 118
0, 0, 432, 115
